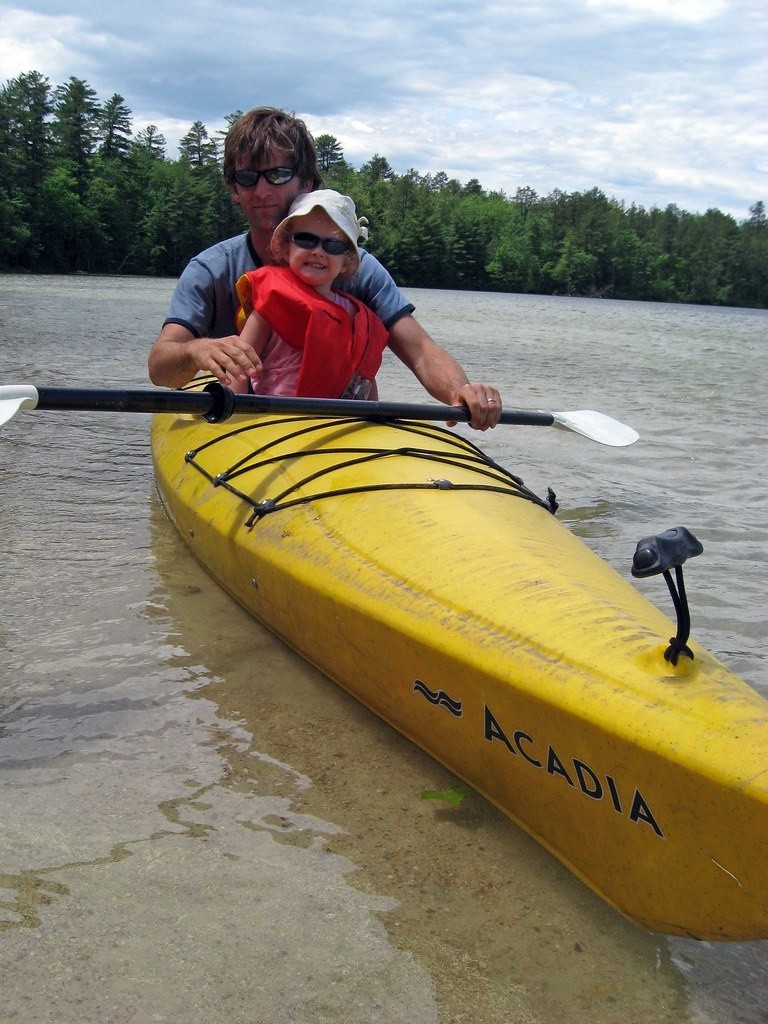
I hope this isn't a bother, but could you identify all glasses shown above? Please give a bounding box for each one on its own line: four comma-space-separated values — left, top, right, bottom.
235, 165, 298, 187
288, 231, 350, 255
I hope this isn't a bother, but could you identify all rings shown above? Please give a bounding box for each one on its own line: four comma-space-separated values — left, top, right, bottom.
486, 398, 496, 403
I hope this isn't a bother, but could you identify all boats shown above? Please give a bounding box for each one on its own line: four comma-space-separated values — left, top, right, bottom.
150, 369, 767, 943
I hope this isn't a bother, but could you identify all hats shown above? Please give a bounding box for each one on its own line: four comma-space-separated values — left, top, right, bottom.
271, 189, 369, 278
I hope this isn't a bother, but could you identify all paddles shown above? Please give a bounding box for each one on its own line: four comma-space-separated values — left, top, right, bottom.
0, 380, 641, 449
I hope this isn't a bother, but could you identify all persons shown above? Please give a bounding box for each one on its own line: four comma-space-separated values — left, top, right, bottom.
148, 109, 502, 432
225, 188, 390, 396
